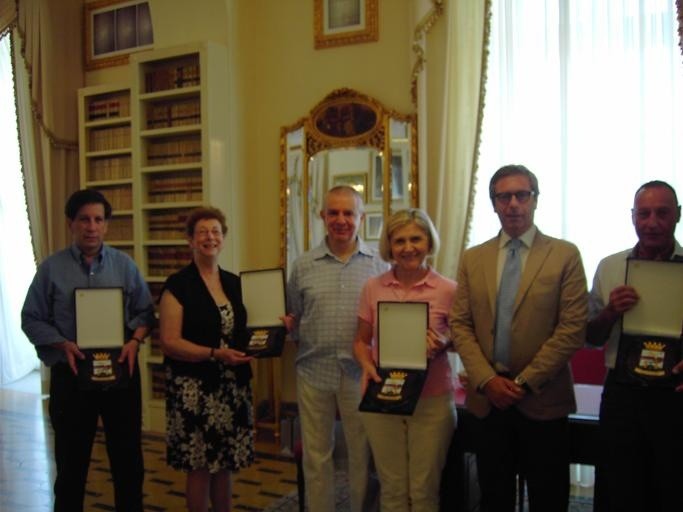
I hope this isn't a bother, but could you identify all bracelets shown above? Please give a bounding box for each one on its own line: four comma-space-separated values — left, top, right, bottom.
210, 347, 216, 363
130, 336, 144, 345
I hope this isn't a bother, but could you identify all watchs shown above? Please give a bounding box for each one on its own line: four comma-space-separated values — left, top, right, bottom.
289, 314, 297, 321
514, 375, 530, 394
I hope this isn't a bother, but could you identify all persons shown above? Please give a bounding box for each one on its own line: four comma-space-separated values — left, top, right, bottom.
451, 164, 590, 510
19, 189, 157, 511
588, 180, 682, 510
289, 185, 390, 511
353, 207, 464, 511
158, 208, 296, 511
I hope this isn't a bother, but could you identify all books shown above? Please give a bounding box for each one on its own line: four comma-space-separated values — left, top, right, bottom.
82, 89, 134, 257
150, 56, 201, 398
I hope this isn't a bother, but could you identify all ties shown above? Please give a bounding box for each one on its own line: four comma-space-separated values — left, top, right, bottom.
494, 239, 523, 376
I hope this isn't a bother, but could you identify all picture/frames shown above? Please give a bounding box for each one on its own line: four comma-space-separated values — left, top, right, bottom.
369, 149, 405, 203
388, 117, 412, 145
82, 0, 154, 72
363, 212, 392, 242
328, 174, 368, 205
312, 1, 380, 50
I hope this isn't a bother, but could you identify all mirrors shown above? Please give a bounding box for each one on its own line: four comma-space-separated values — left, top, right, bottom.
278, 87, 419, 278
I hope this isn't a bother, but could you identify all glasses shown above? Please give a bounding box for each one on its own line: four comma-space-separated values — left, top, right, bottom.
491, 188, 536, 205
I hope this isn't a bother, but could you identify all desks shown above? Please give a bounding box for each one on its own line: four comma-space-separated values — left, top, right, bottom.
450, 385, 605, 467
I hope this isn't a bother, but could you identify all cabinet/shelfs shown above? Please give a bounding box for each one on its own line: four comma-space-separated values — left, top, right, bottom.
128, 37, 237, 440
74, 80, 134, 272
